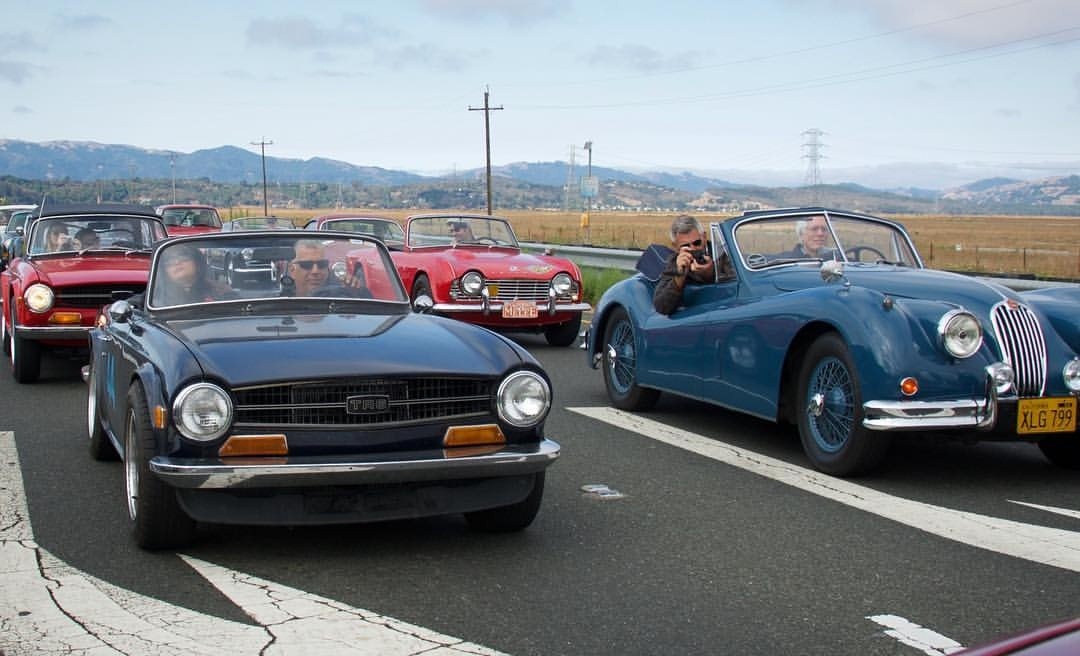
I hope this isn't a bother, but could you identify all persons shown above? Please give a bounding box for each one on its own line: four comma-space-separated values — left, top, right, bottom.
126, 245, 238, 309
258, 240, 376, 298
652, 215, 736, 318
770, 215, 845, 261
447, 219, 495, 247
73, 230, 97, 250
45, 223, 78, 251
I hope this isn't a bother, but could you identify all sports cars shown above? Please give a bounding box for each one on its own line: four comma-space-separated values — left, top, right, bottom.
344, 213, 593, 347
582, 206, 1080, 478
86, 229, 561, 555
0, 200, 414, 383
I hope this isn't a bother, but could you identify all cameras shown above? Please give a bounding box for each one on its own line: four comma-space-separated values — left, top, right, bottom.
687, 250, 706, 266
63, 236, 72, 243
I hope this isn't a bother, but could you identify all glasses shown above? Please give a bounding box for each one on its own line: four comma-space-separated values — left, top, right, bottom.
678, 234, 703, 249
449, 228, 462, 234
162, 253, 194, 265
293, 259, 329, 270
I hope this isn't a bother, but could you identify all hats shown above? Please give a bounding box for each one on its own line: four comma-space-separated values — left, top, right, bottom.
447, 217, 470, 226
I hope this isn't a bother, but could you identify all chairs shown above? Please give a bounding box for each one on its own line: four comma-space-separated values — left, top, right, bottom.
164, 216, 176, 224
